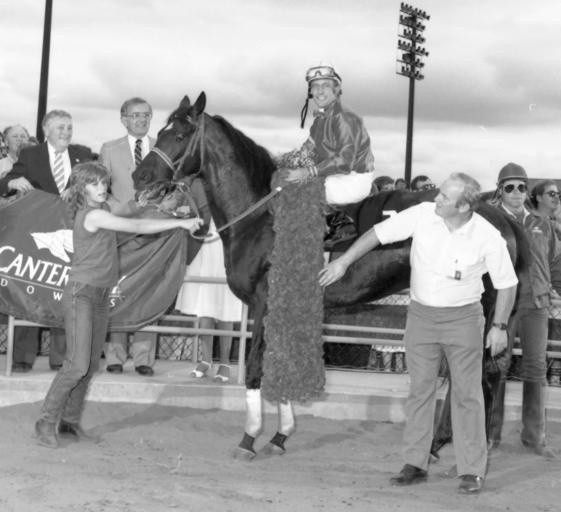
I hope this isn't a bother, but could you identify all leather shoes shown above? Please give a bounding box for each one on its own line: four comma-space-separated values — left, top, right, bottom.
106, 364, 121, 372
135, 366, 152, 375
11, 362, 31, 373
389, 464, 427, 486
51, 364, 61, 370
458, 475, 481, 494
34, 419, 58, 449
57, 420, 99, 445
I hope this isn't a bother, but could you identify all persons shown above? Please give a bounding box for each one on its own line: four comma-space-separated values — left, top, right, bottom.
35, 161, 204, 448
316, 173, 518, 492
531, 180, 560, 386
283, 65, 374, 248
0, 110, 98, 372
0, 124, 30, 206
483, 163, 558, 457
98, 98, 159, 375
365, 176, 436, 374
175, 217, 243, 383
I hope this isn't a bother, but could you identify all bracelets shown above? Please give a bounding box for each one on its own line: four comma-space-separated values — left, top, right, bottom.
492, 322, 507, 330
128, 200, 142, 215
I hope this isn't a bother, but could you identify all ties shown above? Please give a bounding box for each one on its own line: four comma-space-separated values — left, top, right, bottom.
134, 139, 141, 166
53, 152, 64, 192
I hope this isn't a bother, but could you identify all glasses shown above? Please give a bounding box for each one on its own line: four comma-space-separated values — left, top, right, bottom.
418, 183, 435, 191
126, 113, 152, 120
542, 191, 560, 197
305, 66, 342, 81
502, 184, 526, 193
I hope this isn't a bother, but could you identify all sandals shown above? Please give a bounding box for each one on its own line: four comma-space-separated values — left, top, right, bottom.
190, 360, 211, 379
213, 364, 229, 383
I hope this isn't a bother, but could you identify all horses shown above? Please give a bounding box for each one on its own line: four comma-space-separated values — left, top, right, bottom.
131, 91, 531, 462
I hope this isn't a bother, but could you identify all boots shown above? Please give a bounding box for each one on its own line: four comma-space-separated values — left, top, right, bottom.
521, 381, 558, 458
484, 379, 504, 451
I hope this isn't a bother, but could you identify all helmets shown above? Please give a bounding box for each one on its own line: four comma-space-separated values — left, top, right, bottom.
308, 72, 341, 92
498, 162, 527, 182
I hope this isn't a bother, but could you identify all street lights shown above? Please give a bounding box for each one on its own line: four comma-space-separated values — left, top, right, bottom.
397, 2, 431, 189
